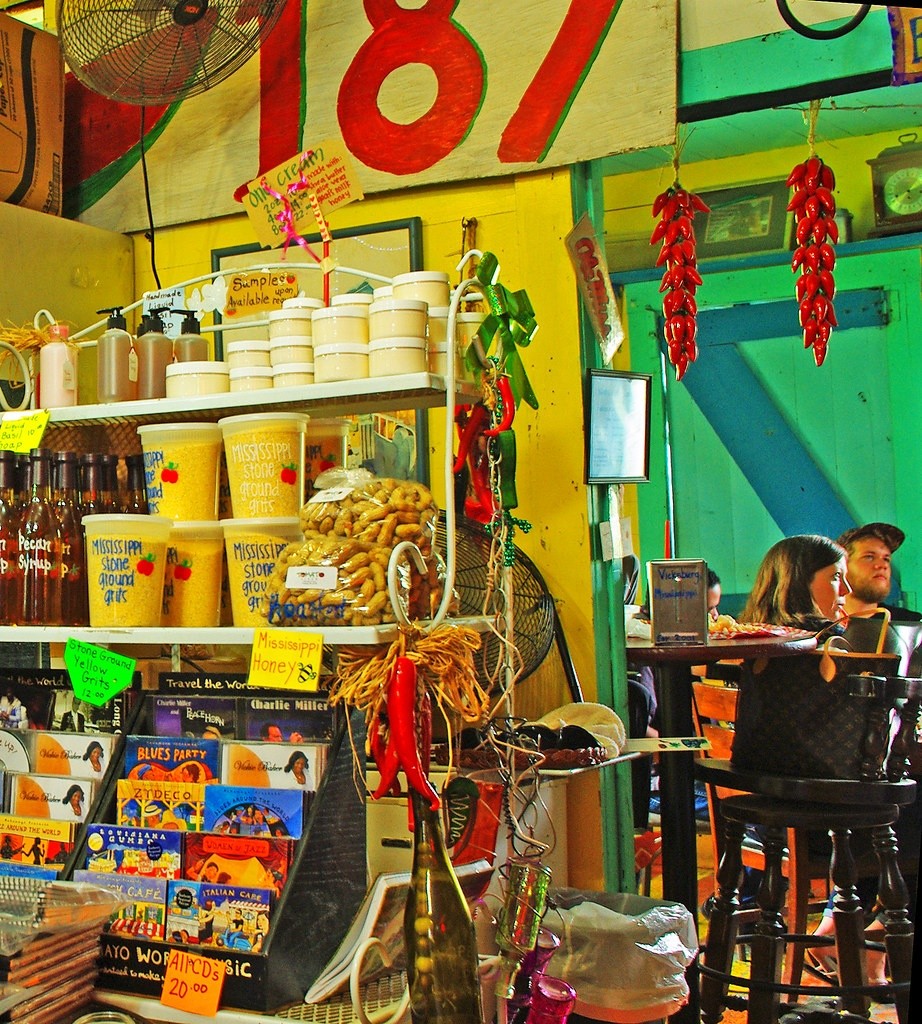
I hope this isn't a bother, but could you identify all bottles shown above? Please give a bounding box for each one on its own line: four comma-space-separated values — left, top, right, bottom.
507, 925, 577, 1024
493, 863, 551, 1000
40, 325, 80, 408
0, 449, 149, 627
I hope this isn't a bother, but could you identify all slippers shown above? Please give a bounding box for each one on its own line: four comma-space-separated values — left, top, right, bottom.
867, 978, 892, 1003
801, 944, 839, 985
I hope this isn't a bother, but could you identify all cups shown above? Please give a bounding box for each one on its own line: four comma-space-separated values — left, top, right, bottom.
82, 412, 354, 627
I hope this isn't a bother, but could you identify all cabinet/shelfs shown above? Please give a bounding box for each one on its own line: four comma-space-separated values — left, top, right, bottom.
365, 759, 567, 891
0, 247, 501, 1024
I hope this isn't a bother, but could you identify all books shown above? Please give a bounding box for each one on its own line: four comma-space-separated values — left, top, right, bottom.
0, 687, 339, 955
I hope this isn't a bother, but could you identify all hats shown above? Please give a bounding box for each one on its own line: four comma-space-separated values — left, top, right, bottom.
836, 522, 905, 553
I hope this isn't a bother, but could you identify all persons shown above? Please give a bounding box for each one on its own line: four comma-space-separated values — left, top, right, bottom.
61, 696, 84, 733
261, 724, 302, 742
731, 522, 922, 1004
0, 688, 21, 728
628, 566, 764, 918
57, 785, 88, 823
80, 741, 107, 779
277, 751, 313, 790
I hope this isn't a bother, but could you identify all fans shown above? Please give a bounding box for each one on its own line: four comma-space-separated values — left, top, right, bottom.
321, 508, 556, 705
43, 1, 288, 106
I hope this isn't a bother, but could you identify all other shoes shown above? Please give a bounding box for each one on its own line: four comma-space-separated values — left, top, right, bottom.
701, 891, 787, 954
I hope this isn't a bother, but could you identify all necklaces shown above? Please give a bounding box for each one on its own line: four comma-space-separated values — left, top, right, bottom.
92, 762, 98, 766
74, 806, 79, 810
296, 773, 301, 779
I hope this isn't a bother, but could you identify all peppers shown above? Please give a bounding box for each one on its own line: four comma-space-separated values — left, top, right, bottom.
368, 656, 439, 812
453, 375, 515, 520
649, 188, 711, 382
785, 157, 839, 366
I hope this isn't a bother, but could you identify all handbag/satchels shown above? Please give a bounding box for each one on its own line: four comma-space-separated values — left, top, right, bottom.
729, 608, 902, 780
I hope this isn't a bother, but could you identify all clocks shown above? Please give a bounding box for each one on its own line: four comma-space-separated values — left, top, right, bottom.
871, 155, 922, 228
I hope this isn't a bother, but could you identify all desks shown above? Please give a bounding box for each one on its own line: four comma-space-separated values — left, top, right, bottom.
626, 621, 817, 1024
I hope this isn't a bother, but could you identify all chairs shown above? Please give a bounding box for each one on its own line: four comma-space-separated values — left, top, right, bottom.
693, 620, 922, 1024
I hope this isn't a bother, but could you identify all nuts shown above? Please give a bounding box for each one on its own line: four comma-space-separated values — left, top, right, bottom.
259, 476, 440, 628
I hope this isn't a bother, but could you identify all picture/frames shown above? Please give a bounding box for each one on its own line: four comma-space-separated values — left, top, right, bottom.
689, 174, 794, 264
211, 216, 430, 494
